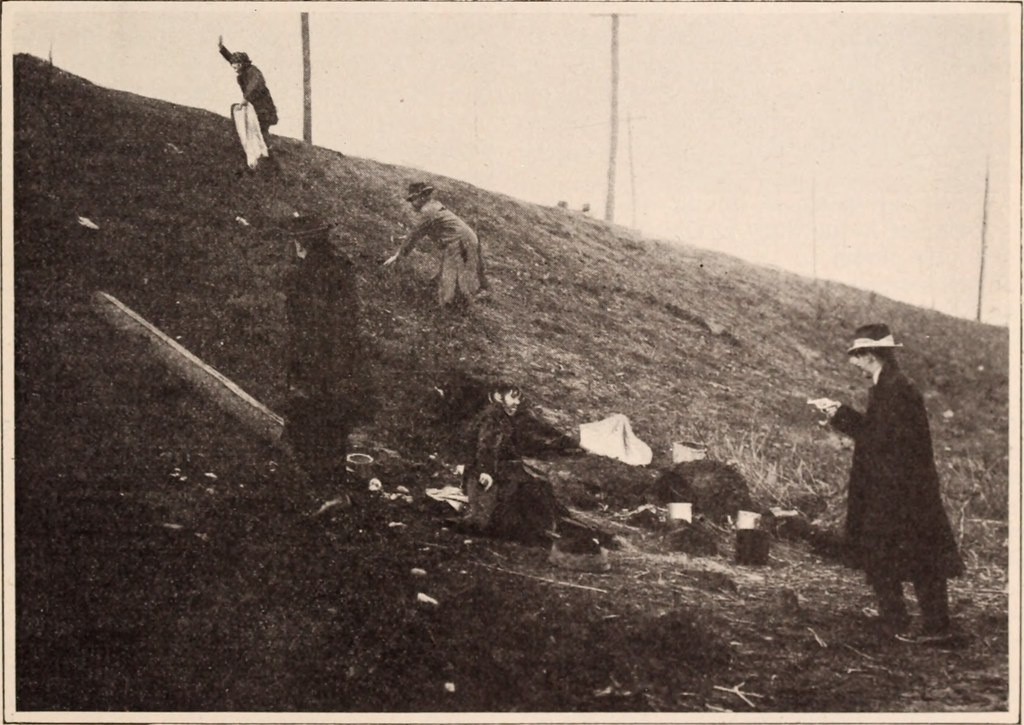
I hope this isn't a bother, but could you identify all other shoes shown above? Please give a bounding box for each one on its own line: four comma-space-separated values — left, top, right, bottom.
895, 629, 953, 642
864, 607, 911, 629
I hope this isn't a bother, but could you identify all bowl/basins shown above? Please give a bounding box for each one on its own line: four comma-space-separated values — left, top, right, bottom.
346, 454, 374, 474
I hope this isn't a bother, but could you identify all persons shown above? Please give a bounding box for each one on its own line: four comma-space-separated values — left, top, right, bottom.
821, 324, 963, 644
466, 381, 572, 547
384, 181, 480, 310
218, 36, 278, 146
281, 214, 363, 482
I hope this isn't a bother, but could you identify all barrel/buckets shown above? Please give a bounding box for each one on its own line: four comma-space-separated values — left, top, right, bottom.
673, 441, 707, 464
736, 510, 761, 529
667, 503, 692, 524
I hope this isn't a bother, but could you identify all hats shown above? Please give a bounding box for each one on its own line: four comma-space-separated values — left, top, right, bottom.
847, 323, 902, 352
288, 213, 333, 236
404, 182, 435, 202
230, 52, 253, 64
